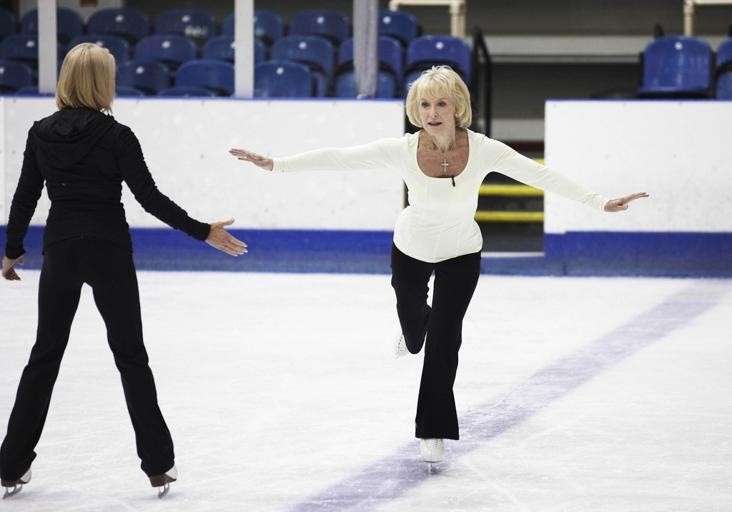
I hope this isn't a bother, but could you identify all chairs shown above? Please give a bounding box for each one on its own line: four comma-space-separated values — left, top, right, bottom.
712, 38, 732, 102
636, 34, 714, 98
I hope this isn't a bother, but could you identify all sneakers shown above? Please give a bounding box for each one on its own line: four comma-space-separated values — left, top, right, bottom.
398, 334, 410, 357
1, 468, 31, 486
421, 439, 444, 463
149, 466, 177, 486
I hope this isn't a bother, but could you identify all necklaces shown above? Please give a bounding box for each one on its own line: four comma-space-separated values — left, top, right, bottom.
441, 145, 454, 173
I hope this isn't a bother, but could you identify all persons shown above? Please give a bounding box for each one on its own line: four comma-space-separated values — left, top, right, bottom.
229, 64, 650, 464
0, 42, 248, 488
